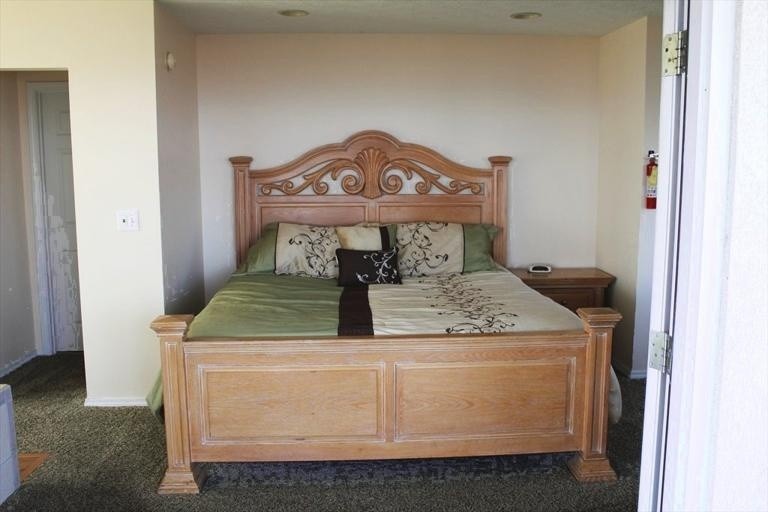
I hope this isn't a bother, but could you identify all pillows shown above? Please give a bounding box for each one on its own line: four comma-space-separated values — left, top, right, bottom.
228, 218, 387, 281
334, 245, 403, 288
333, 225, 397, 251
389, 221, 504, 281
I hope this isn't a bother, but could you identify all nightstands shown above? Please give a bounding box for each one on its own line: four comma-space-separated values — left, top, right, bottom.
506, 265, 617, 317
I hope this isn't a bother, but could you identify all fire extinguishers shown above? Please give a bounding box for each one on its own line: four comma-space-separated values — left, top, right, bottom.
646, 153, 658, 209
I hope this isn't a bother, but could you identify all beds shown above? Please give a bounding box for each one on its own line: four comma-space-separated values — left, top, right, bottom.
146, 128, 622, 499
